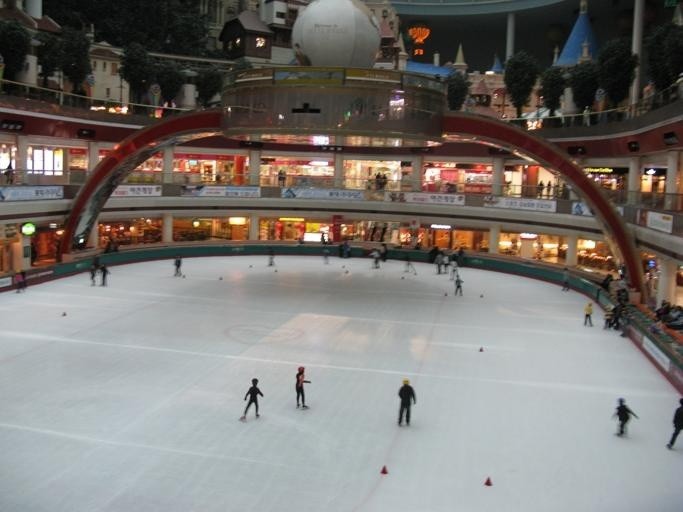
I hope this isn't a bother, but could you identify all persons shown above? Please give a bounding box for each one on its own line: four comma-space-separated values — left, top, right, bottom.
238, 378, 263, 421
609, 396, 639, 438
396, 377, 417, 426
666, 399, 682, 449
295, 366, 311, 409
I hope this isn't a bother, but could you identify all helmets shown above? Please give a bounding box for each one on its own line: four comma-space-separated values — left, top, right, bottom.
251, 378, 258, 385
297, 367, 304, 373
402, 377, 409, 385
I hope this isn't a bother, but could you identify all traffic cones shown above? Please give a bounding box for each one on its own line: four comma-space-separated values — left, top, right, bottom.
378, 464, 388, 476
483, 477, 492, 487
477, 345, 485, 354
